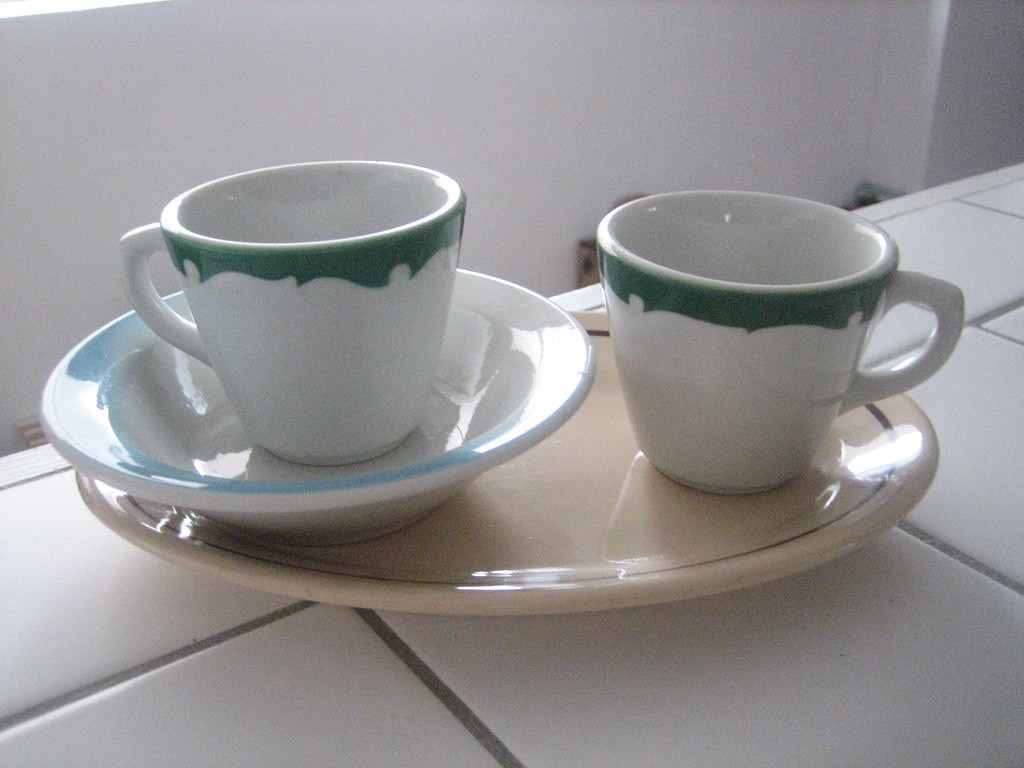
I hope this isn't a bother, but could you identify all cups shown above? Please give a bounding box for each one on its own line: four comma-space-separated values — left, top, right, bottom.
115, 158, 468, 466
593, 191, 965, 494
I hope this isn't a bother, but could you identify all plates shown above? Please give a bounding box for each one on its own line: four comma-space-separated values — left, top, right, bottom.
42, 266, 596, 547
77, 311, 942, 617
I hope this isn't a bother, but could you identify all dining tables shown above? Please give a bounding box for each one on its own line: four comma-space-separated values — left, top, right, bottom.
0, 162, 1023, 767
